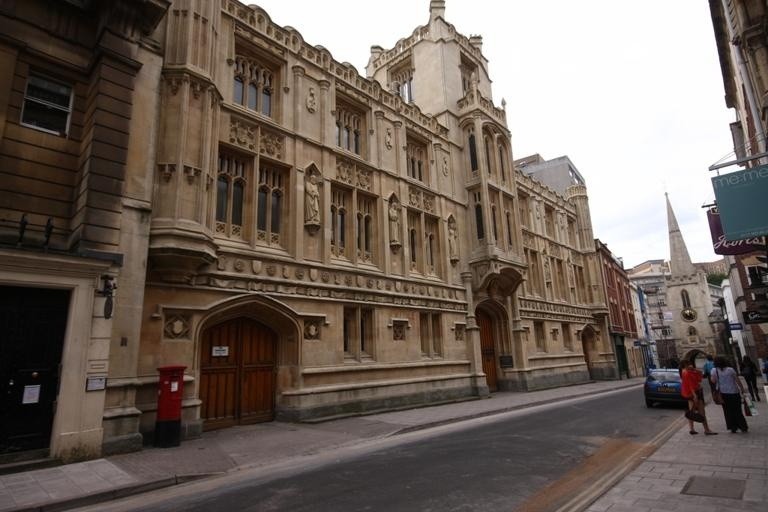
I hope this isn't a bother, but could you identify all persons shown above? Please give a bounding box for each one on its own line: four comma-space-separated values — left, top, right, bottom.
567, 262, 574, 286
739, 354, 760, 402
703, 356, 721, 405
448, 222, 458, 257
389, 202, 400, 243
678, 359, 719, 435
306, 87, 318, 112
710, 357, 749, 433
305, 175, 321, 222
385, 127, 393, 147
686, 361, 705, 404
543, 259, 551, 280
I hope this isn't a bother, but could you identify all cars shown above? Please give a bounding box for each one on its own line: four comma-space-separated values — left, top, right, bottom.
643, 367, 690, 411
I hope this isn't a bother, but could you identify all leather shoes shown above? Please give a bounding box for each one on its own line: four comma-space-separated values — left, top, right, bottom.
689, 431, 697, 435
704, 431, 718, 435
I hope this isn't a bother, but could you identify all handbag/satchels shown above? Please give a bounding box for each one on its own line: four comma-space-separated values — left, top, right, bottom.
742, 396, 758, 417
684, 401, 706, 423
711, 390, 724, 405
